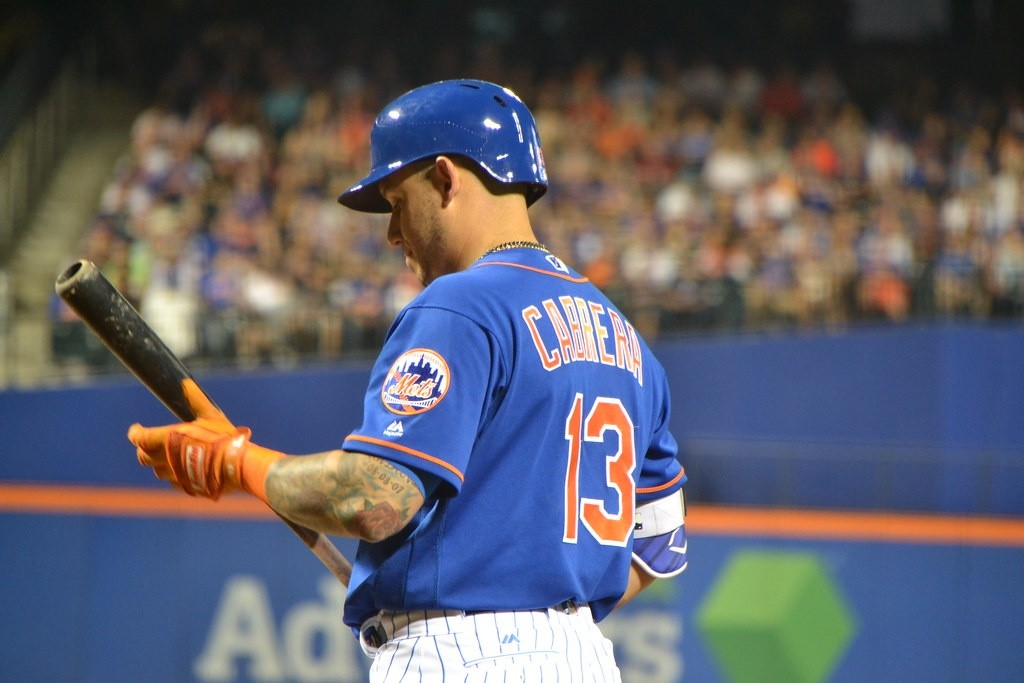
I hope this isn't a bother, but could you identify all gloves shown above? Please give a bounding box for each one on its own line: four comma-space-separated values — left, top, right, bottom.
127, 379, 251, 503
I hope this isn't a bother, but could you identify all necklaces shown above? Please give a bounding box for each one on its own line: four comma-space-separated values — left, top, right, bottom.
478, 241, 550, 261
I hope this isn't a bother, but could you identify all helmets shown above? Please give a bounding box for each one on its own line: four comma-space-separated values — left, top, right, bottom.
335, 78, 549, 213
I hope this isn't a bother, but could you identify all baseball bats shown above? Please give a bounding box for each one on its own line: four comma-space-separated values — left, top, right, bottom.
55, 259, 353, 589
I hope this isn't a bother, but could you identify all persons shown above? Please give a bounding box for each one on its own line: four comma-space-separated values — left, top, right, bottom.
127, 78, 688, 683
48, 42, 1023, 374
